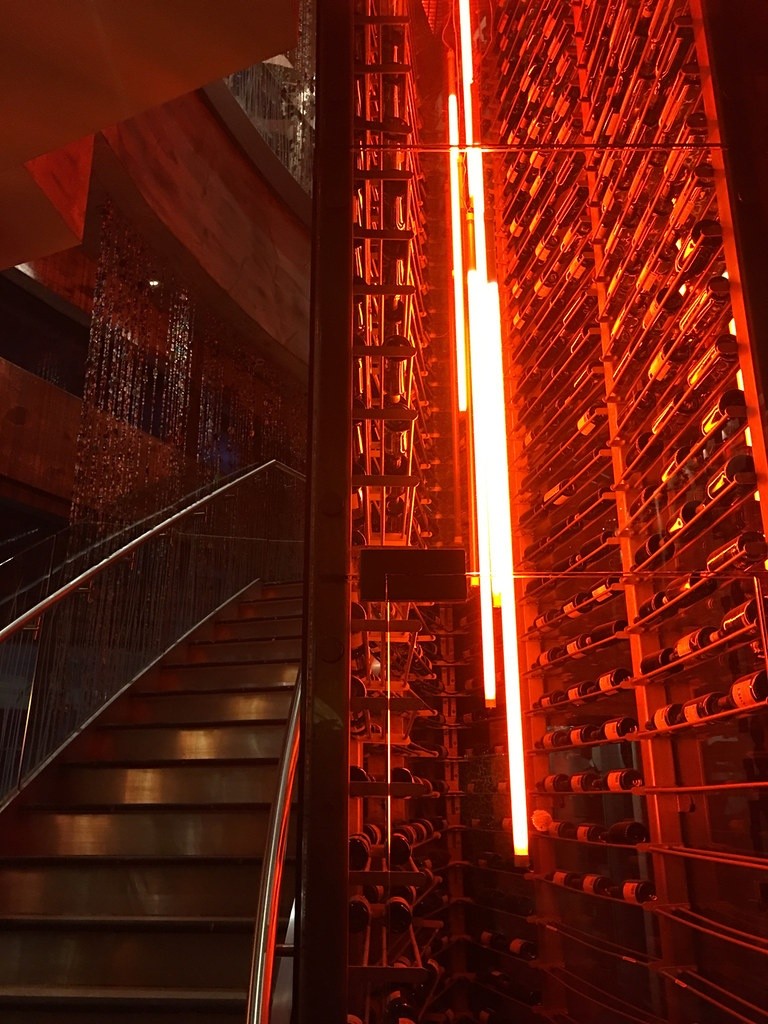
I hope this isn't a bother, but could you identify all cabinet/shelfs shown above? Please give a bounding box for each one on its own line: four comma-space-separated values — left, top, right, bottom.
293, 0, 768, 1024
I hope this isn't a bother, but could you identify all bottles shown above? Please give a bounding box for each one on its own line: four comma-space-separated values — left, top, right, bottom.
347, 0, 768, 1024
